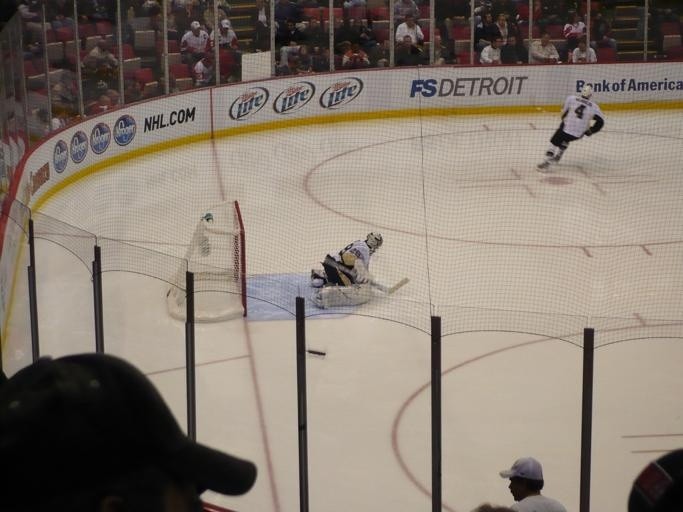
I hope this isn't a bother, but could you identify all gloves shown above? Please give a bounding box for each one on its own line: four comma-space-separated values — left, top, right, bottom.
354, 258, 370, 285
584, 128, 593, 136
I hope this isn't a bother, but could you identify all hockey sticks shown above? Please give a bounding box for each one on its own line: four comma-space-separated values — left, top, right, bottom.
325, 259, 410, 294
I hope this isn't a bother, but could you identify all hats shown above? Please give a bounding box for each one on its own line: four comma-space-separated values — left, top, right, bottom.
221, 19, 231, 29
190, 20, 200, 31
498, 457, 544, 481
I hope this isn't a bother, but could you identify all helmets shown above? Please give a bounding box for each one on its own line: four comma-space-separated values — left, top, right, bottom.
365, 231, 384, 251
582, 85, 593, 97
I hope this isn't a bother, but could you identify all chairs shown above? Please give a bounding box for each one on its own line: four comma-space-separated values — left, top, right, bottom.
20, 1, 682, 120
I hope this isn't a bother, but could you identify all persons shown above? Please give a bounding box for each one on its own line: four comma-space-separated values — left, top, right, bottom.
310, 231, 384, 309
498, 457, 567, 512
474, 2, 683, 66
537, 85, 606, 171
176, 2, 471, 88
2, 1, 180, 138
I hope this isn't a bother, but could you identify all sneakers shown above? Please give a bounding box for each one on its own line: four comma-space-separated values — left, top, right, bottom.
537, 151, 561, 170
